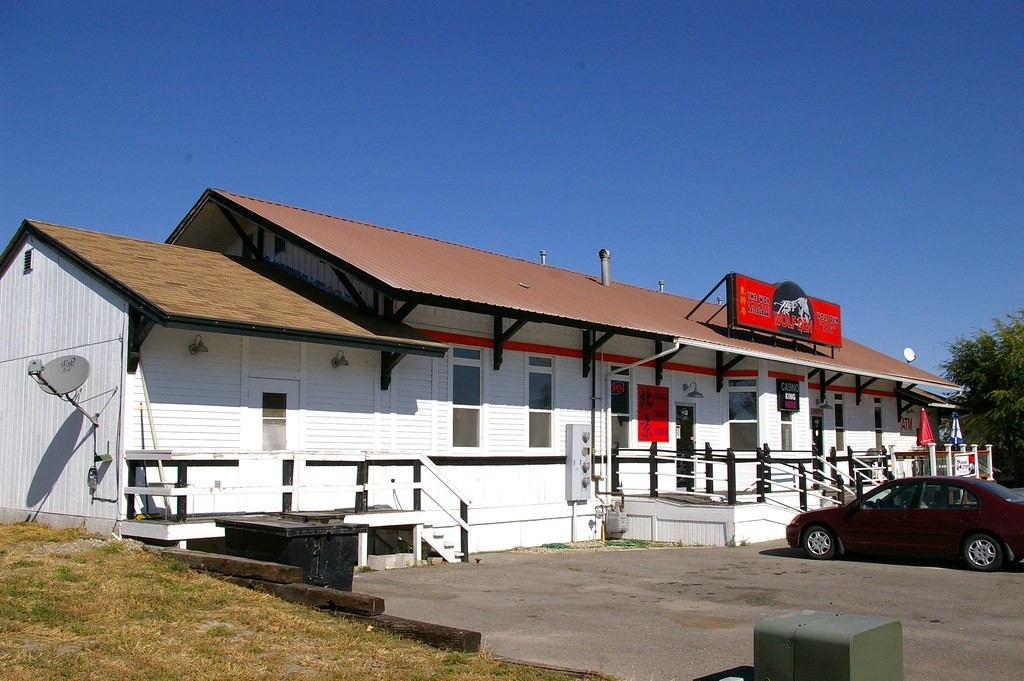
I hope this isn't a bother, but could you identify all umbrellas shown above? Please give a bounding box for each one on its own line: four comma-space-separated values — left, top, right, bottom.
917, 408, 935, 451
949, 412, 963, 451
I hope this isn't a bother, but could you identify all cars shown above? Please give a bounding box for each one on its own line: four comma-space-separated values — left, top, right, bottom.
786, 475, 1024, 573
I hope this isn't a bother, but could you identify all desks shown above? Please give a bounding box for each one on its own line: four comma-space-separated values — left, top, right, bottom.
213, 518, 369, 592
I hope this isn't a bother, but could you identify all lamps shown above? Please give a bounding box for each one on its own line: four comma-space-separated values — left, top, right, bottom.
816, 398, 833, 409
190, 335, 209, 355
907, 409, 915, 414
683, 382, 704, 399
331, 350, 349, 368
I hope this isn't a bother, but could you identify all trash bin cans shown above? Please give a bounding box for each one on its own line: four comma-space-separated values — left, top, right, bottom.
215, 511, 370, 592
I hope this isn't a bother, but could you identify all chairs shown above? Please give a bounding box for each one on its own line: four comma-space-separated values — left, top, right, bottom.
928, 491, 945, 508
